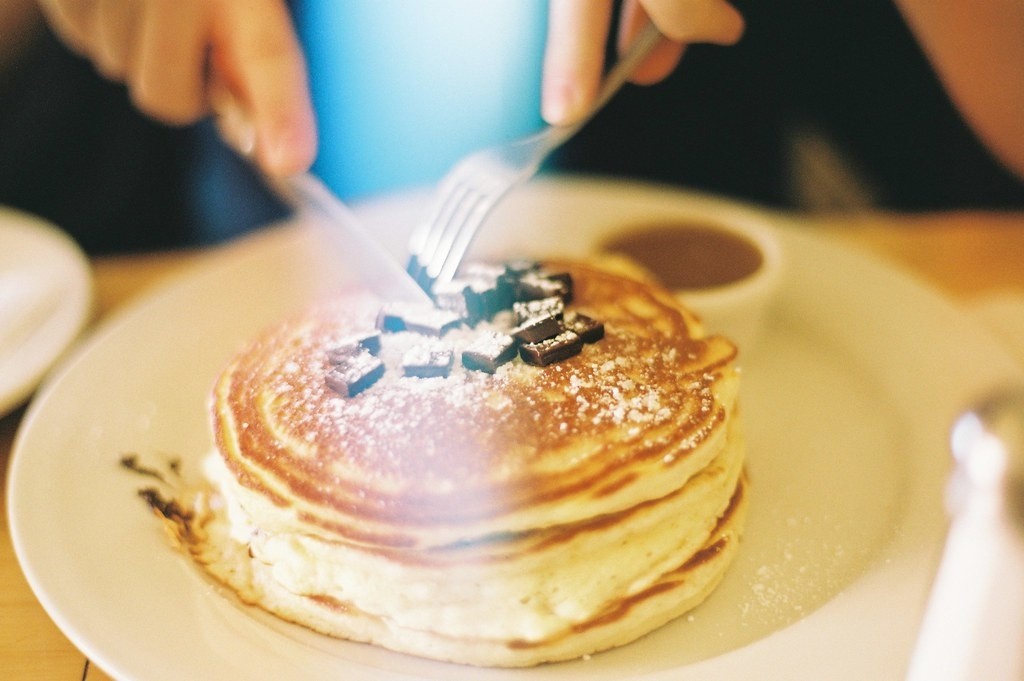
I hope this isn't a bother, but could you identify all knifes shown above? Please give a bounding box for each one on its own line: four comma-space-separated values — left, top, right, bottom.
207, 94, 445, 331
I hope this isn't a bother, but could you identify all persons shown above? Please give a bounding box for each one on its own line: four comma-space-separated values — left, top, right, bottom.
0, 0, 1024, 255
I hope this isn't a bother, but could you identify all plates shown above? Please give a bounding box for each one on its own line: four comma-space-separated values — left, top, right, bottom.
0, 202, 99, 421
0, 162, 1024, 681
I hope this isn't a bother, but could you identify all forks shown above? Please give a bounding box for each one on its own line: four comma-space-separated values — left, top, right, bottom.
403, 21, 688, 303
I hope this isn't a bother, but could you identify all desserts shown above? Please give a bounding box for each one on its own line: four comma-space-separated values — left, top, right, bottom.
200, 253, 752, 668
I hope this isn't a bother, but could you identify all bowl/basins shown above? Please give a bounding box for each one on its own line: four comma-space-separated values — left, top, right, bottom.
588, 204, 787, 385
